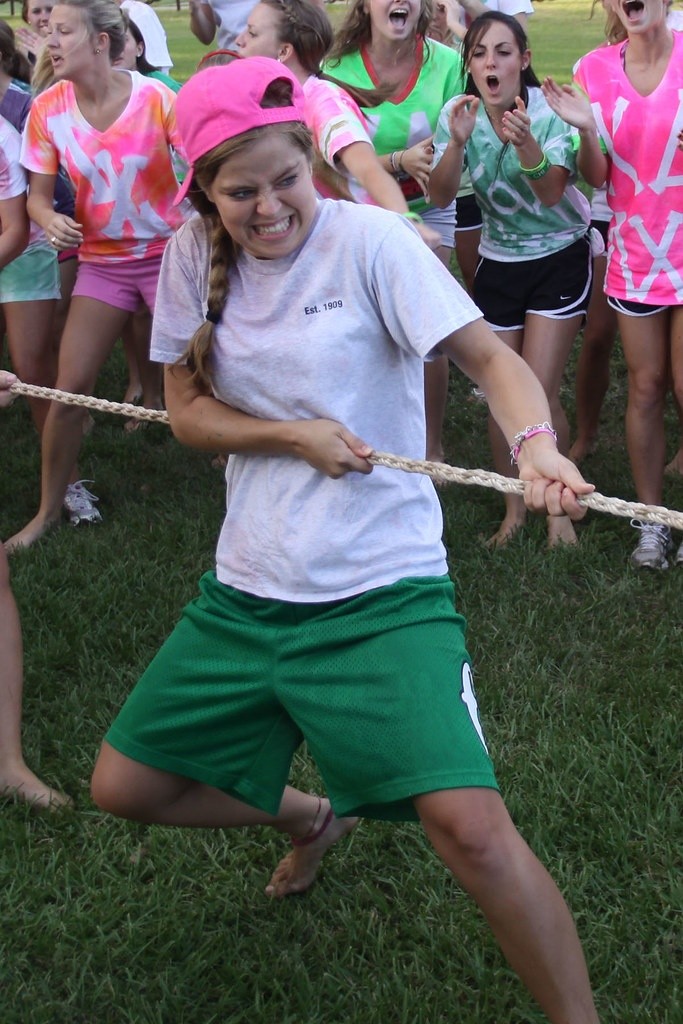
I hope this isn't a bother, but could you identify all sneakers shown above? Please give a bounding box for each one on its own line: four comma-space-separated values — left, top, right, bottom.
629, 518, 672, 569
62, 479, 102, 527
676, 542, 683, 564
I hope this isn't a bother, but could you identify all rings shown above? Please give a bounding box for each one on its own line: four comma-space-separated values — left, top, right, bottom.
50, 236, 57, 245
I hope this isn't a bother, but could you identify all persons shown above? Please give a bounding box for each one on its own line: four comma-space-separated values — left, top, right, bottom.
88, 55, 598, 1023
426, 9, 595, 555
0, 0, 536, 552
541, 0, 683, 571
0, 369, 77, 813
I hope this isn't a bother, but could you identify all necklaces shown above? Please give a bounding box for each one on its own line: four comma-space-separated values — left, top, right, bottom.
484, 103, 507, 129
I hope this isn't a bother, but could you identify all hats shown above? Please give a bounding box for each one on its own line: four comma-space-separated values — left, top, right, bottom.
170, 57, 307, 207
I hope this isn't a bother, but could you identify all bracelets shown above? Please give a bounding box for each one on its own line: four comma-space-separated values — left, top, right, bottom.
510, 420, 557, 466
402, 210, 424, 224
399, 147, 408, 174
519, 150, 552, 180
389, 151, 397, 172
